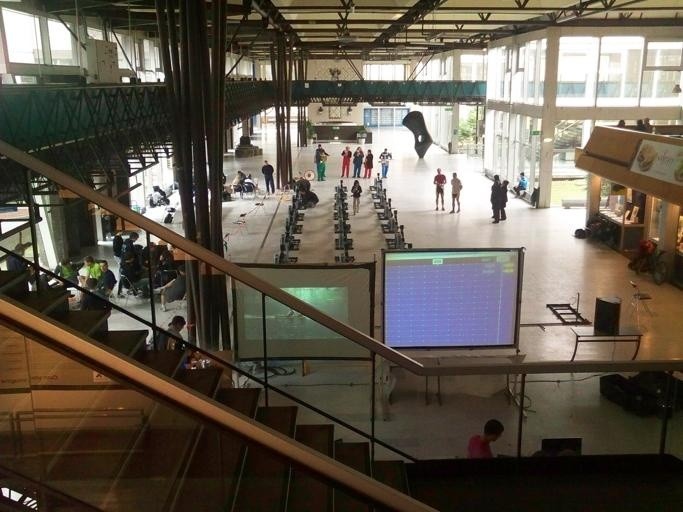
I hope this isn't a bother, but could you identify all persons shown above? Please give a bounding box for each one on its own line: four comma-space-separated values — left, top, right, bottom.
318, 150, 327, 181
293, 177, 310, 201
158, 315, 186, 354
151, 185, 167, 206
449, 173, 462, 213
514, 172, 527, 196
262, 160, 274, 195
434, 168, 446, 211
298, 187, 319, 208
468, 419, 504, 459
222, 171, 256, 203
341, 147, 392, 179
314, 144, 330, 169
6, 229, 186, 311
617, 117, 651, 133
351, 180, 362, 215
491, 175, 509, 223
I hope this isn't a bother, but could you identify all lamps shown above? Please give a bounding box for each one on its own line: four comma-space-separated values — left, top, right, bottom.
346, 106, 354, 115
318, 107, 324, 114
225, 6, 554, 64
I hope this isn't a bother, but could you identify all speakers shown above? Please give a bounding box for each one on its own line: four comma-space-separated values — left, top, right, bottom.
594, 296, 622, 336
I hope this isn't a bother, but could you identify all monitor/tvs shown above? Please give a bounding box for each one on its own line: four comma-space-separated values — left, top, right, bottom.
541, 437, 582, 457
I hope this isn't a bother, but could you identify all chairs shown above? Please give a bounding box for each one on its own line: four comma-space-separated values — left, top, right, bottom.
225, 181, 258, 199
25, 233, 190, 320
626, 282, 656, 321
222, 193, 269, 247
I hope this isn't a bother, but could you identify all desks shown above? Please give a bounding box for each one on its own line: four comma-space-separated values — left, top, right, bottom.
568, 324, 644, 363
